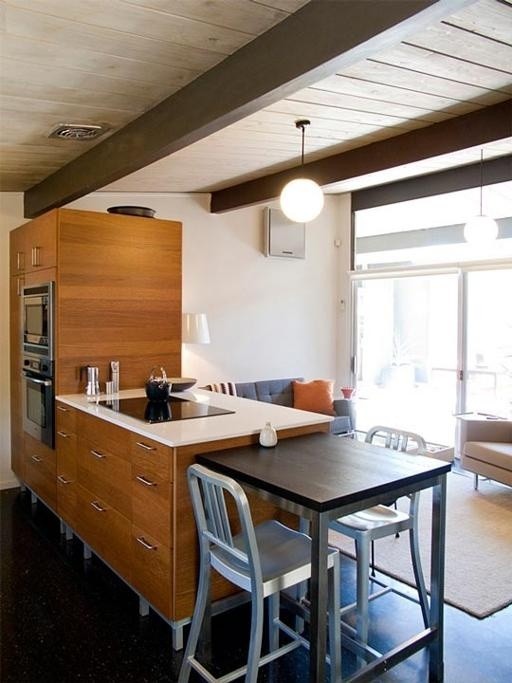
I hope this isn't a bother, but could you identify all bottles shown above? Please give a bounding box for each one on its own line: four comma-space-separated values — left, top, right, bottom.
259, 421, 277, 447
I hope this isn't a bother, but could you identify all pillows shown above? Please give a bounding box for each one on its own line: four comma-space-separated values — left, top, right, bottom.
291, 378, 337, 415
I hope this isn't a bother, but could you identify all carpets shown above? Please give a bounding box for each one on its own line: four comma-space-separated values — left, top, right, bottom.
326, 470, 512, 620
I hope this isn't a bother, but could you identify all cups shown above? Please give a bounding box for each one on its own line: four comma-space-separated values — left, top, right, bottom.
105, 380, 114, 395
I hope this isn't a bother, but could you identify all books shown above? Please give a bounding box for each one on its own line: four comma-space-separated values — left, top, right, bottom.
96, 366, 100, 394
91, 366, 96, 396
78, 366, 91, 395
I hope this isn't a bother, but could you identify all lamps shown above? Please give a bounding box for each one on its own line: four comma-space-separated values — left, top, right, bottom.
279, 119, 325, 225
180, 312, 211, 345
464, 147, 498, 247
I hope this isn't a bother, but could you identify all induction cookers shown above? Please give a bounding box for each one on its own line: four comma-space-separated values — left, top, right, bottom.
88, 392, 236, 425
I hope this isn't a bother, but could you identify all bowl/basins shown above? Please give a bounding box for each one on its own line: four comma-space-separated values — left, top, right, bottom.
166, 377, 197, 393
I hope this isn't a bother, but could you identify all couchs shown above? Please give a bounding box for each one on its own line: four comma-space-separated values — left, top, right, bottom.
198, 377, 358, 440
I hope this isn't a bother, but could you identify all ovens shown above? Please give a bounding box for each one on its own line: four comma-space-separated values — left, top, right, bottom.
19, 281, 56, 451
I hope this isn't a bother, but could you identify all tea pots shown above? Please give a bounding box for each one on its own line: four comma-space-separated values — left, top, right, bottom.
80, 365, 100, 396
143, 364, 172, 401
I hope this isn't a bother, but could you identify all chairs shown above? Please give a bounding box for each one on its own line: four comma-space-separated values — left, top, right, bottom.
459, 416, 512, 491
178, 465, 343, 683
295, 426, 424, 670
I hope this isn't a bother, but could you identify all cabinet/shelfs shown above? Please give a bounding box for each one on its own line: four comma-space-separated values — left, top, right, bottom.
55, 401, 76, 532
21, 433, 55, 508
76, 411, 129, 580
129, 423, 333, 622
10, 275, 22, 475
10, 209, 57, 278
